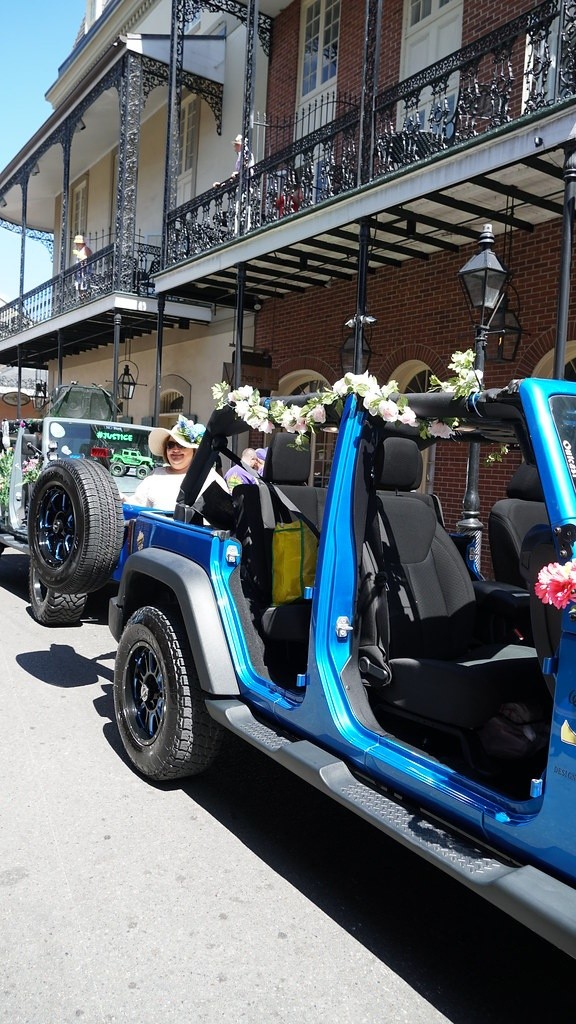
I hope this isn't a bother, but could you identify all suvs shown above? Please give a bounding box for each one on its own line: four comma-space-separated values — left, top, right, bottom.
0, 419, 170, 626
27, 378, 576, 960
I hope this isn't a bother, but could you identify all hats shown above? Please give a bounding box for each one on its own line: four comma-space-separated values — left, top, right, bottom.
148, 415, 206, 457
231, 135, 247, 147
74, 236, 86, 243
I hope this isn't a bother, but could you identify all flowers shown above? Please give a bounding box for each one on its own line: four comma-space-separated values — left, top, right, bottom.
0, 418, 44, 509
534, 558, 576, 613
176, 414, 204, 445
211, 349, 485, 450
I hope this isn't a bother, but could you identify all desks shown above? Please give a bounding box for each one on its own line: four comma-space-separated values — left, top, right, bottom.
133, 271, 148, 296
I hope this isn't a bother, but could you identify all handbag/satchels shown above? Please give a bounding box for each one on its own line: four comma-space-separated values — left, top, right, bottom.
271, 519, 317, 606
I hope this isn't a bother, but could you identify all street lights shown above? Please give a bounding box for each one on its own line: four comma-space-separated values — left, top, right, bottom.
456, 224, 509, 574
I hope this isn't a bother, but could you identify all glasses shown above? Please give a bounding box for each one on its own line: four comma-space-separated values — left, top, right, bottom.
252, 458, 258, 461
165, 441, 186, 449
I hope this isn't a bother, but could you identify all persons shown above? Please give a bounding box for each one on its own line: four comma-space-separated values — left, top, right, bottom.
120, 417, 229, 515
213, 134, 253, 236
224, 447, 269, 491
276, 185, 305, 218
72, 235, 93, 300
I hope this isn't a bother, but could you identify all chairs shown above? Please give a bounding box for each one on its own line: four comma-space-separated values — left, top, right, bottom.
230, 432, 563, 794
141, 255, 161, 295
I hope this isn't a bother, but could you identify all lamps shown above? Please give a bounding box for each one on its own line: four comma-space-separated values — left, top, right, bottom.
27, 383, 50, 410
34, 164, 40, 173
106, 323, 147, 400
77, 120, 86, 130
484, 184, 532, 364
0, 197, 7, 207
